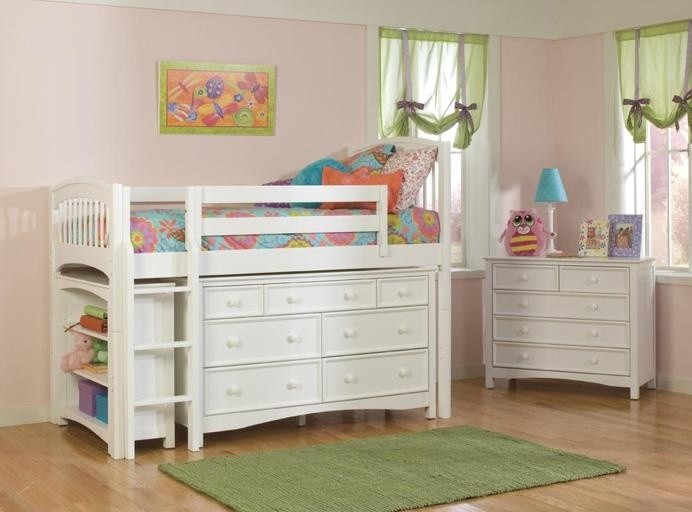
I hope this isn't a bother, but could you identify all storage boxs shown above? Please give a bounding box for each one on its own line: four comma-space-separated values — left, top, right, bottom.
78, 380, 106, 417
95, 392, 108, 424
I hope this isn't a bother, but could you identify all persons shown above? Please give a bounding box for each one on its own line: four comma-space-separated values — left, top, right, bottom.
586, 226, 601, 249
615, 225, 633, 249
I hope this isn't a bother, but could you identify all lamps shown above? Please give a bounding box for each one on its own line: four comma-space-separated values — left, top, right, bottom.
534, 168, 569, 257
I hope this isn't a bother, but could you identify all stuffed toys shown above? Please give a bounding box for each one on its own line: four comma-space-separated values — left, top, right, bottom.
498, 207, 557, 257
60, 333, 94, 374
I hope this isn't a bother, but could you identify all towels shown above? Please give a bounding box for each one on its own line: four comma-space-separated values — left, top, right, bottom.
80, 304, 107, 333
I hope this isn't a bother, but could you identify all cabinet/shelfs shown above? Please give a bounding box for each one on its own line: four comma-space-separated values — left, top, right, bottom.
481, 257, 658, 399
55, 274, 176, 459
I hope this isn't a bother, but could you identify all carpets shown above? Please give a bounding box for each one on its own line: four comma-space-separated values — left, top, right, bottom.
157, 424, 628, 512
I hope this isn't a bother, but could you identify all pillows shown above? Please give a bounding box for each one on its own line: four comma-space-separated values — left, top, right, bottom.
253, 143, 438, 214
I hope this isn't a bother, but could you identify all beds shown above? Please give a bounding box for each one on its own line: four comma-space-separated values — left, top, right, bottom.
48, 137, 452, 459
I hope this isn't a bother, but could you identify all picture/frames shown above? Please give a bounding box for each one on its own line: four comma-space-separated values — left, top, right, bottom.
157, 61, 277, 135
608, 214, 642, 257
577, 218, 608, 256
